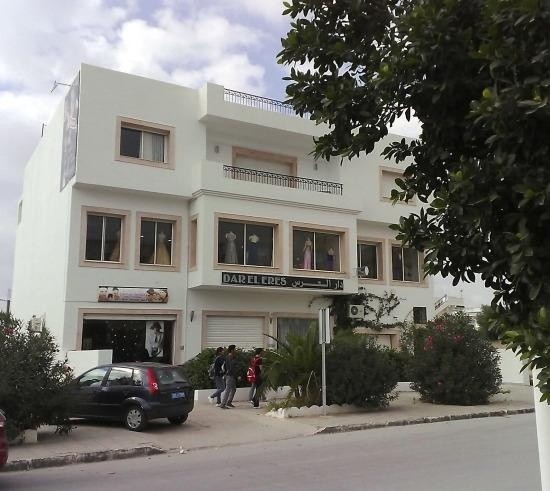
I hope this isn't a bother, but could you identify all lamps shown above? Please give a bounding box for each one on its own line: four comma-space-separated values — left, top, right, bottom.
356, 266, 369, 276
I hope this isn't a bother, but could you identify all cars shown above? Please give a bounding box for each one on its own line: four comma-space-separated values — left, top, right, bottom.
63, 362, 197, 432
0, 408, 9, 471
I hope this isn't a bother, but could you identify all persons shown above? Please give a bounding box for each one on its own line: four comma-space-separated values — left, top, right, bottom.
149, 322, 164, 357
110, 231, 121, 262
144, 288, 168, 302
224, 231, 238, 264
208, 347, 226, 407
248, 233, 258, 265
303, 237, 313, 269
248, 348, 268, 403
220, 345, 241, 409
328, 248, 335, 270
251, 351, 265, 408
99, 287, 120, 301
149, 232, 171, 265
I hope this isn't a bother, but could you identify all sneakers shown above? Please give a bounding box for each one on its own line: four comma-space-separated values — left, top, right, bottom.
208, 395, 234, 409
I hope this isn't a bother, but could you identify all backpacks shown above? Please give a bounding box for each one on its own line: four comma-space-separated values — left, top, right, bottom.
247, 367, 256, 382
208, 363, 216, 380
217, 359, 226, 375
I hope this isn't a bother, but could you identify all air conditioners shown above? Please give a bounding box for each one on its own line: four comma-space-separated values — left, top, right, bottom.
347, 305, 364, 318
29, 318, 43, 333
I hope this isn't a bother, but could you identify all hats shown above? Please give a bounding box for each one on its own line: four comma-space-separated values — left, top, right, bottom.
151, 322, 161, 328
